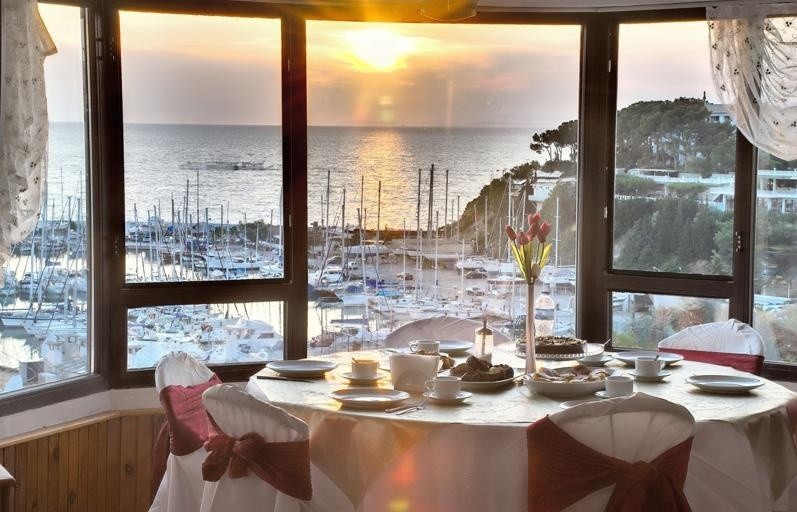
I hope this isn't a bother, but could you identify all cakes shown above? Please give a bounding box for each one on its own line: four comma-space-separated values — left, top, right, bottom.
516, 336, 584, 353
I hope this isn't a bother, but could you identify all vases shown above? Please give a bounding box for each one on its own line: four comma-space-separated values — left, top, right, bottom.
524, 285, 536, 373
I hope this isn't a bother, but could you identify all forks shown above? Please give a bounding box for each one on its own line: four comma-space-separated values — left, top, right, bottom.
385, 399, 427, 415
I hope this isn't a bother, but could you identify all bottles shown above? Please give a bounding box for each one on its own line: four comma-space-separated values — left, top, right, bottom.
534, 290, 554, 336
474, 319, 494, 364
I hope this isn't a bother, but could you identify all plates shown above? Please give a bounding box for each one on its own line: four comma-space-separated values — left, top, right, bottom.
434, 365, 526, 388
611, 349, 684, 366
575, 354, 612, 366
420, 390, 474, 405
339, 371, 386, 383
328, 387, 409, 409
683, 373, 765, 395
560, 400, 593, 410
264, 358, 338, 377
594, 388, 639, 401
409, 338, 474, 353
626, 368, 671, 380
522, 368, 623, 397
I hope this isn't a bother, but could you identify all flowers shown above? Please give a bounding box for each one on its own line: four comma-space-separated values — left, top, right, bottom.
503, 213, 554, 322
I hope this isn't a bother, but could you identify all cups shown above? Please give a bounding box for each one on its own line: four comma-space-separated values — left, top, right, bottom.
605, 375, 632, 396
409, 340, 440, 355
634, 355, 664, 375
350, 357, 380, 376
582, 342, 605, 360
425, 376, 462, 396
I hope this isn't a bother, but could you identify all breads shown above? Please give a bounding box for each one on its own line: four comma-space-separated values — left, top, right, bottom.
439, 355, 513, 381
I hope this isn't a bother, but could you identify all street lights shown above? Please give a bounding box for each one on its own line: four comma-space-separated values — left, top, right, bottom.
782, 281, 791, 298
761, 283, 769, 297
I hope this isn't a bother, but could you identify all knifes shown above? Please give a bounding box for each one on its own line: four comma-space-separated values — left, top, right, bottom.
256, 373, 313, 383
